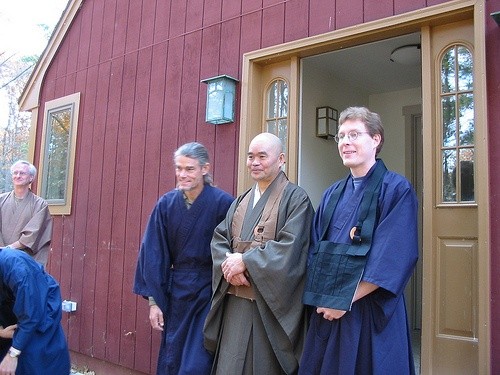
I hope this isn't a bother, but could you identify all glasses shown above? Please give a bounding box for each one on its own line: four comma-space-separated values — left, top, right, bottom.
332, 130, 369, 143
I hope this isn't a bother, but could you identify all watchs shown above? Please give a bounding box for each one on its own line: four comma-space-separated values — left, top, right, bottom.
8, 349, 20, 359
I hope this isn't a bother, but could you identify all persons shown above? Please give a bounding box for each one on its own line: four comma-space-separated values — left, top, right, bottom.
132, 142, 235, 375
452, 161, 474, 201
298, 106, 418, 375
0, 160, 53, 273
203, 133, 315, 375
0, 247, 72, 375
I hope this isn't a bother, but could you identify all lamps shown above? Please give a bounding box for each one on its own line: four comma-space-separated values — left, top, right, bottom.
316, 106, 337, 140
391, 44, 421, 69
200, 74, 240, 125
